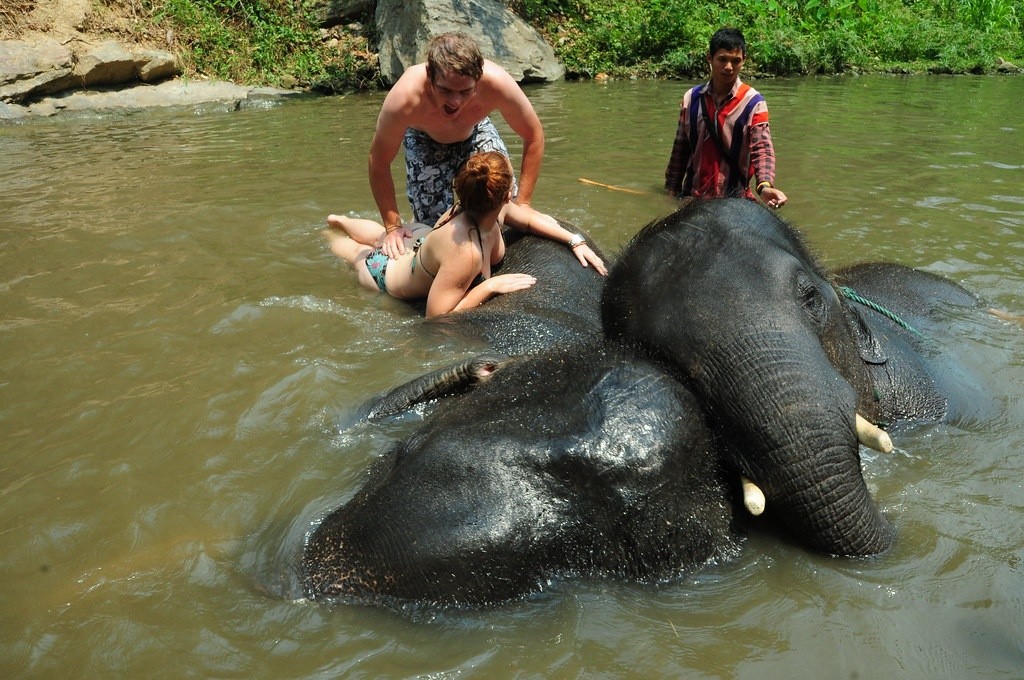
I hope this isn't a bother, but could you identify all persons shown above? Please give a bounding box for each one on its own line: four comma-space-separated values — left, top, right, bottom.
368, 32, 544, 260
664, 28, 787, 208
329, 151, 608, 319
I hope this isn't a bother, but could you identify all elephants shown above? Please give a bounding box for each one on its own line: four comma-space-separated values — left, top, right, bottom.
604, 198, 1004, 557
229, 214, 733, 624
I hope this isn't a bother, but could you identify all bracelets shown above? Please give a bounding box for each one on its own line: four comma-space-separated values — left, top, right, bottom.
568, 234, 586, 249
386, 226, 402, 235
757, 182, 772, 195
